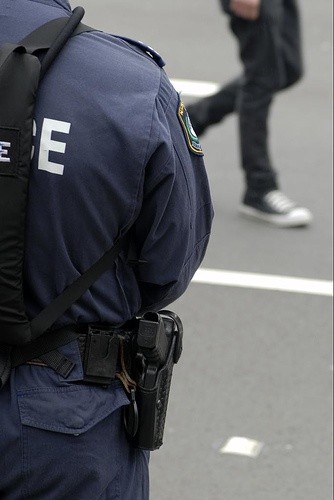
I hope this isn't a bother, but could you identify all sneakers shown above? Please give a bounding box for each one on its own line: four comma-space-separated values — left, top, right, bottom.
241, 189, 312, 226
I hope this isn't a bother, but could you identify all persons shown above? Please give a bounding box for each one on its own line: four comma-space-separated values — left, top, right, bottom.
184, 0, 317, 230
0, 0, 215, 500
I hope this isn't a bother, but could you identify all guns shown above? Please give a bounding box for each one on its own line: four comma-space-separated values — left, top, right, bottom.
139, 310, 184, 452
